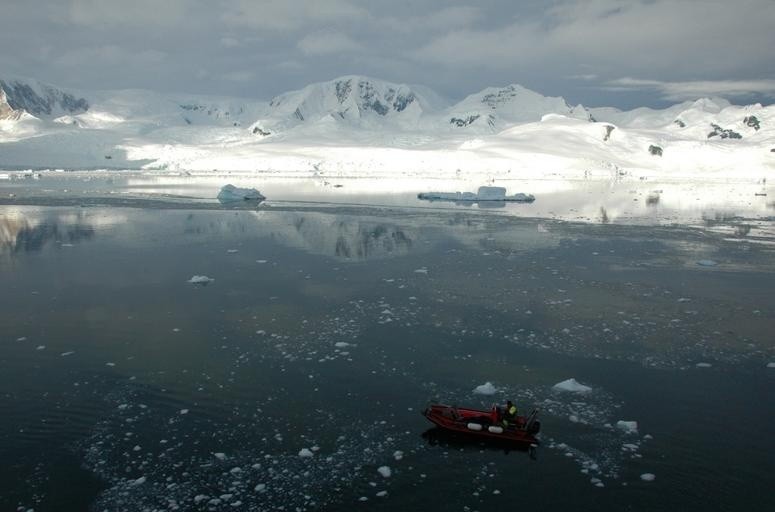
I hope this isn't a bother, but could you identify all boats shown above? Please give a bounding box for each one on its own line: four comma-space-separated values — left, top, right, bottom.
423, 402, 542, 447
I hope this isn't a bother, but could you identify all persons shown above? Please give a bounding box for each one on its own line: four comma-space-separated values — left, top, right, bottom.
503, 401, 518, 421
